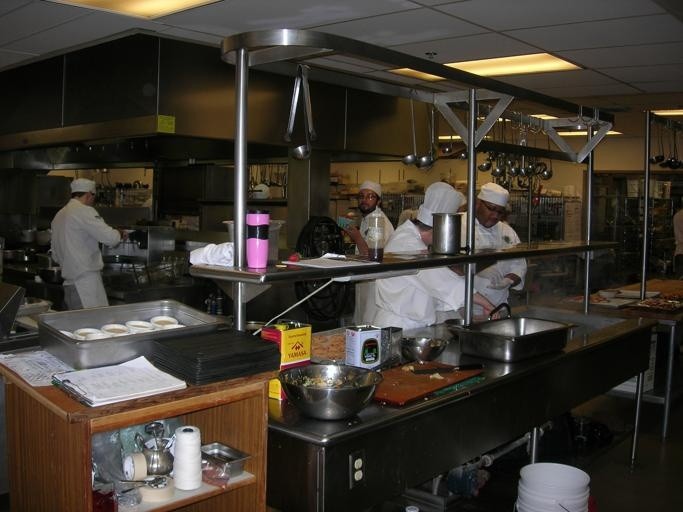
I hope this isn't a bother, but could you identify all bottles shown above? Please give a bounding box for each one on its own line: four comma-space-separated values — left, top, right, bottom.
368, 205, 385, 261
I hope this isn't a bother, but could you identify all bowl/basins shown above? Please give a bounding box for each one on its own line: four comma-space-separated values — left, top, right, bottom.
73, 327, 101, 338
36, 299, 232, 370
150, 315, 179, 327
126, 320, 154, 331
277, 363, 383, 420
200, 440, 252, 478
396, 336, 450, 361
598, 290, 618, 299
452, 316, 581, 363
101, 323, 129, 335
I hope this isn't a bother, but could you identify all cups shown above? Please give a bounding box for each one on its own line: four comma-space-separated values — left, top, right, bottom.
429, 212, 463, 256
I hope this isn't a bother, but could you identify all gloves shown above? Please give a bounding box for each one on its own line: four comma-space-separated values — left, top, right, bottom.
487, 279, 511, 290
477, 267, 502, 284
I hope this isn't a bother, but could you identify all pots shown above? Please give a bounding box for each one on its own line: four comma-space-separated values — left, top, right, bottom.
444, 303, 511, 340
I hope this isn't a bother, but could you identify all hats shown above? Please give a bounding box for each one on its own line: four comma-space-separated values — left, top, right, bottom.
478, 182, 509, 207
417, 182, 465, 227
360, 181, 381, 196
71, 179, 96, 192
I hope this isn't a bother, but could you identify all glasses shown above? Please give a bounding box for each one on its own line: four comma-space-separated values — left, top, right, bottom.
359, 193, 378, 201
483, 201, 506, 213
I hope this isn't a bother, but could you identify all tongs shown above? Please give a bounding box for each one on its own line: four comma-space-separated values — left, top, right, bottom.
282, 63, 316, 143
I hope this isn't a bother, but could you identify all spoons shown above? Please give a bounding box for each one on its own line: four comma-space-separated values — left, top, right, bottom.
649, 122, 683, 170
456, 110, 469, 161
415, 105, 436, 171
401, 97, 419, 165
477, 110, 553, 181
144, 422, 162, 449
441, 129, 452, 154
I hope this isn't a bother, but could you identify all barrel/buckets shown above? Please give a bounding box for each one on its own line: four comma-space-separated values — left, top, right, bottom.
514, 461, 592, 511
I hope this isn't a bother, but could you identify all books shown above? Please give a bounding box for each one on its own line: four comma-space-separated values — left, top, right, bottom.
54, 354, 186, 408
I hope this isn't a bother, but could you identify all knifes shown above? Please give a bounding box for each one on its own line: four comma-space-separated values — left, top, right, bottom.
411, 363, 485, 374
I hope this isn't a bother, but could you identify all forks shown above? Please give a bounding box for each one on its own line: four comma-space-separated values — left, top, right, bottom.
289, 67, 312, 162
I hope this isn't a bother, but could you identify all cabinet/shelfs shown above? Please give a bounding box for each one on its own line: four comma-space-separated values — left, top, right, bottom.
190, 242, 619, 330
273, 312, 649, 512
1, 348, 271, 512
574, 281, 683, 440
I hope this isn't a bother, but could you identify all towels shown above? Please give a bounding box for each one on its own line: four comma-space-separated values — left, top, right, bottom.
190, 242, 234, 266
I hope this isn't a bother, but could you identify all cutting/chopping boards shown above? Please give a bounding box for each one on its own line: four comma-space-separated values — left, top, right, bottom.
372, 362, 484, 409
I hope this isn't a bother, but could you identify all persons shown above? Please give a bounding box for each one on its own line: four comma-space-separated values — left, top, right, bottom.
353, 182, 393, 327
52, 178, 128, 309
672, 196, 683, 280
458, 183, 525, 318
369, 182, 500, 332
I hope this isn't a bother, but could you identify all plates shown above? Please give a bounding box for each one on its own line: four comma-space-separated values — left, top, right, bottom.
603, 288, 663, 300
625, 298, 682, 314
567, 294, 640, 310
151, 327, 281, 387
